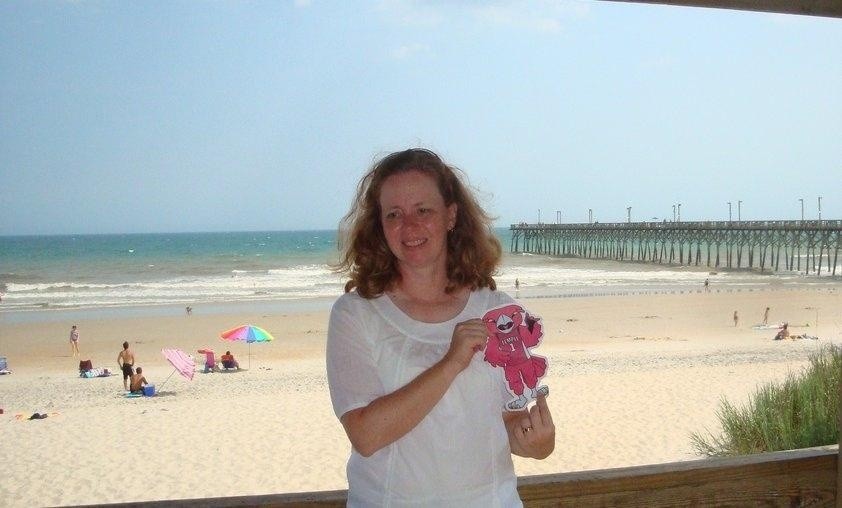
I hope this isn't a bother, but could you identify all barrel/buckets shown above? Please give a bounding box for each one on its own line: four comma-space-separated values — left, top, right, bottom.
144, 384, 156, 396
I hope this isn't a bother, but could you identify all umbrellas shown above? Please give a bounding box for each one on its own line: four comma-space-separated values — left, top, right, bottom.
156, 347, 196, 395
218, 323, 275, 367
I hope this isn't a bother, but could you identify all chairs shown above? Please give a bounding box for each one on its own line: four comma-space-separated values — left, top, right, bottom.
0, 356, 11, 375
222, 360, 240, 370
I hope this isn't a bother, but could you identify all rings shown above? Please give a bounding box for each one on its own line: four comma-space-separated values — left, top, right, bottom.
522, 425, 533, 433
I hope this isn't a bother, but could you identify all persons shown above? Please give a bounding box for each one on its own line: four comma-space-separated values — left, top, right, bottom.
226, 351, 241, 370
129, 367, 148, 393
326, 144, 557, 507
762, 306, 770, 324
776, 323, 790, 339
69, 326, 81, 357
732, 310, 740, 326
515, 277, 520, 289
704, 279, 709, 292
117, 341, 135, 390
